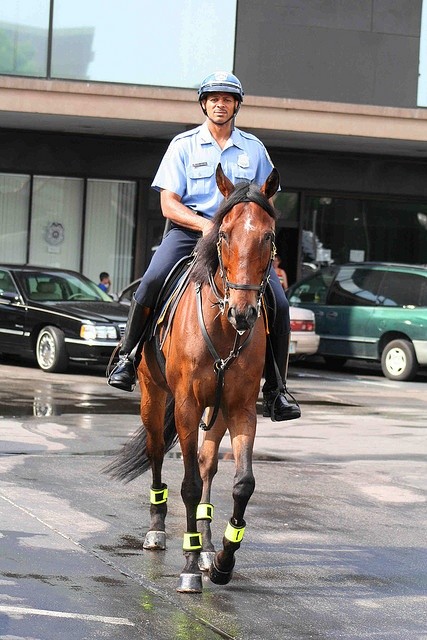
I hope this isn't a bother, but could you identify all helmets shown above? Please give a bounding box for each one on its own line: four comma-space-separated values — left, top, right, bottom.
198, 72, 244, 104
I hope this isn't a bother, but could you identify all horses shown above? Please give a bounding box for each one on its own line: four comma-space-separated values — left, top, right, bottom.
100, 162, 274, 597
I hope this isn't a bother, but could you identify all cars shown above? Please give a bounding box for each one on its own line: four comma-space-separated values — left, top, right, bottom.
1, 263, 131, 373
287, 262, 427, 379
111, 275, 320, 366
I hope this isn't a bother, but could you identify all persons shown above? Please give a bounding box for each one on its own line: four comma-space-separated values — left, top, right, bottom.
95, 272, 111, 302
273, 256, 288, 290
108, 71, 302, 422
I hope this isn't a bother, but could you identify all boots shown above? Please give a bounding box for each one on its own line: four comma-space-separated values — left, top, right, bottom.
261, 330, 301, 421
106, 293, 157, 392
